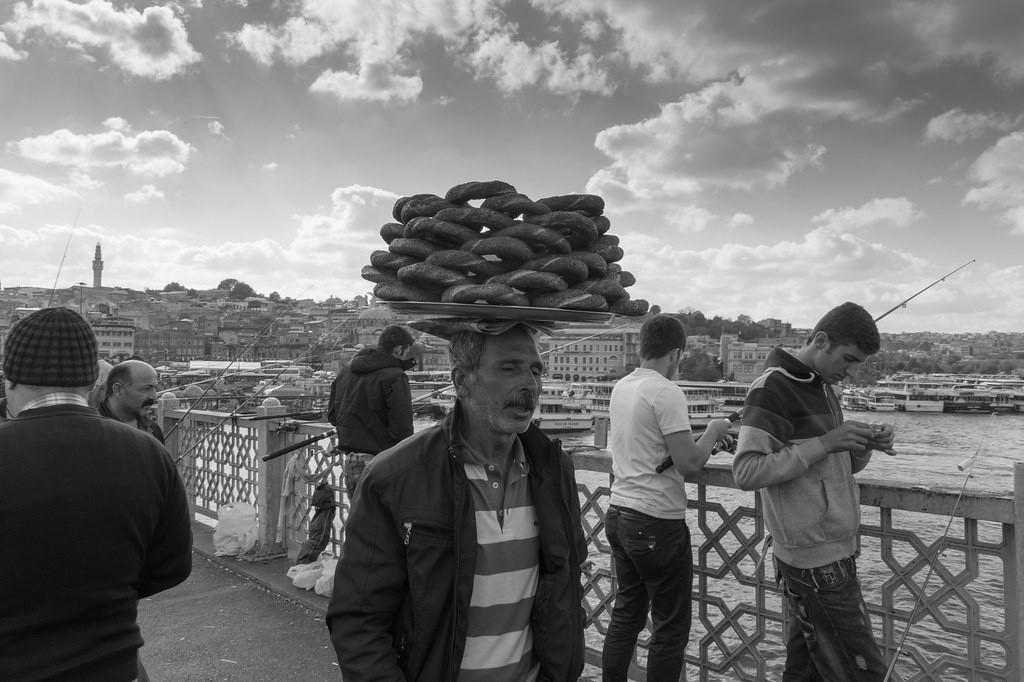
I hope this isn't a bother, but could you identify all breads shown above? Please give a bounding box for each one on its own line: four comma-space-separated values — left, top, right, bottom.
361, 180, 650, 316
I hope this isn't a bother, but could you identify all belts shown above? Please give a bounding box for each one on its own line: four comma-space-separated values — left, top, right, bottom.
347, 455, 373, 461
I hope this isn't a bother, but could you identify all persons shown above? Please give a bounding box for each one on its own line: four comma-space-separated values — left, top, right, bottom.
90, 358, 165, 446
327, 328, 413, 499
599, 315, 733, 682
322, 319, 589, 682
731, 302, 900, 681
0, 304, 194, 682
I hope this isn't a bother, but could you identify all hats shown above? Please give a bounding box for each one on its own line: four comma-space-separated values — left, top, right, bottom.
3, 306, 100, 387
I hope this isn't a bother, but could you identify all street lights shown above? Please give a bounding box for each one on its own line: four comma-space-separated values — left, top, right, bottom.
75, 282, 88, 314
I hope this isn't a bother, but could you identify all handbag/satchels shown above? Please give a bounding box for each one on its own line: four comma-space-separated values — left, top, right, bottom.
287, 550, 340, 597
213, 502, 256, 557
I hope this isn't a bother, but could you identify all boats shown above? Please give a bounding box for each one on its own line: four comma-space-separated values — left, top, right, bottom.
429, 373, 596, 433
867, 396, 896, 411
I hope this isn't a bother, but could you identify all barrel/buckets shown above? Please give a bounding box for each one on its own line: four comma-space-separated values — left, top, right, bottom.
214, 502, 256, 555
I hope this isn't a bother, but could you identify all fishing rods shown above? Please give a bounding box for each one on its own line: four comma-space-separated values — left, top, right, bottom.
155, 281, 655, 467
651, 256, 1001, 682
47, 207, 83, 306
